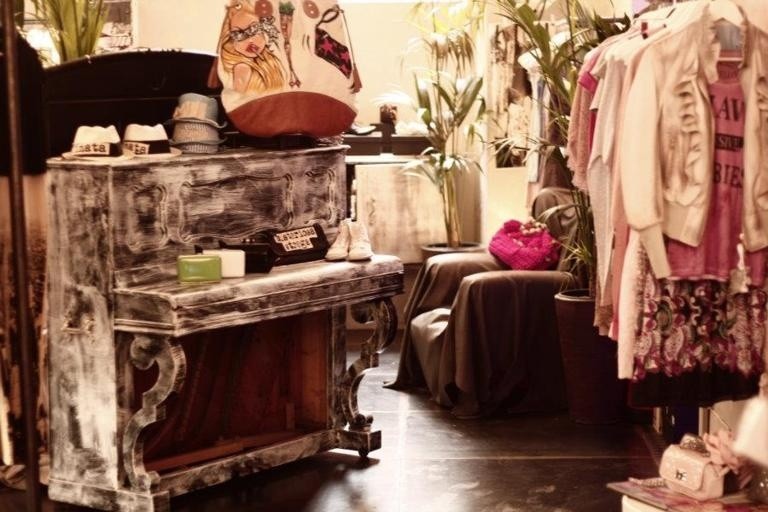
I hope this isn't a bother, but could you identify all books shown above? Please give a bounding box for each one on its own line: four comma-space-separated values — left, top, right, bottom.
607, 479, 768, 510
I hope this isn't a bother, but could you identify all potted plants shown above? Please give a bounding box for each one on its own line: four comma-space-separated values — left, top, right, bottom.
377, 1, 493, 266
490, 0, 634, 427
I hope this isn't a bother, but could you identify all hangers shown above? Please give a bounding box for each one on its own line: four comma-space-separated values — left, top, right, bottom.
604, 0, 747, 64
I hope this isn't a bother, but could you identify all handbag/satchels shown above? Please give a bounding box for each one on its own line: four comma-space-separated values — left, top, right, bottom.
657, 445, 723, 503
258, 223, 331, 264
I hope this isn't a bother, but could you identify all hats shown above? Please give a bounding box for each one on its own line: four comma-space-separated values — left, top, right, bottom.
60, 93, 228, 166
488, 220, 559, 272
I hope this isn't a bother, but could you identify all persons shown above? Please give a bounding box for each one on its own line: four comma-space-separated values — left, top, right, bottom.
221, 0, 286, 93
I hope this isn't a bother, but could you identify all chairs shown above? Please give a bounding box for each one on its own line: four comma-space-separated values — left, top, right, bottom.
385, 187, 593, 421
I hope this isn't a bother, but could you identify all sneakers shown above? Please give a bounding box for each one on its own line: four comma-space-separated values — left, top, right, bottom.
326, 221, 372, 261
